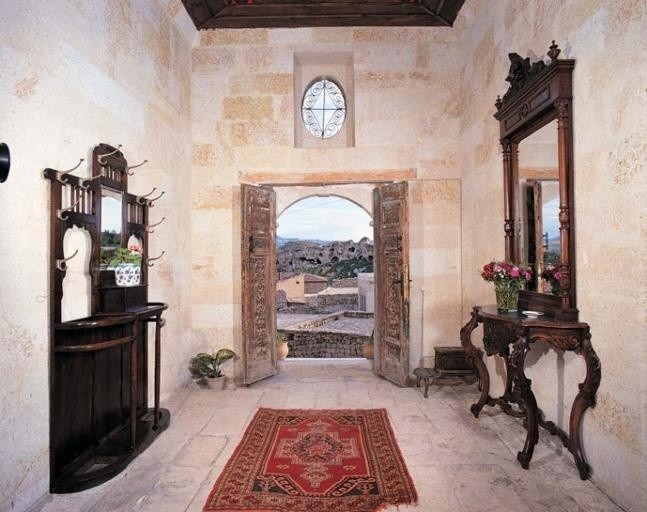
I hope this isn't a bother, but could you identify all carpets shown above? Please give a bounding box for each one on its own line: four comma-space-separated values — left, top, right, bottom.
202, 406, 421, 511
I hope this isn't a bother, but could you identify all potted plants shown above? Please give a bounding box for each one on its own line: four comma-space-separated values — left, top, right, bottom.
363, 329, 376, 359
192, 348, 237, 392
109, 248, 144, 288
276, 335, 290, 361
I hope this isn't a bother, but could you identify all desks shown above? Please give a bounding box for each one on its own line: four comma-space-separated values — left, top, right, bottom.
459, 302, 602, 482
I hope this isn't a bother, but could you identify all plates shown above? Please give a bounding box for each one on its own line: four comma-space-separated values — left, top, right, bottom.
523, 309, 544, 322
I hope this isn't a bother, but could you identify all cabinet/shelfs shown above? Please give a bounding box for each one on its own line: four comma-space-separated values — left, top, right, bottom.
43, 143, 171, 495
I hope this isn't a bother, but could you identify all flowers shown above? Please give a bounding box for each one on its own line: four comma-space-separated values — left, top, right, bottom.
482, 261, 533, 307
540, 263, 561, 296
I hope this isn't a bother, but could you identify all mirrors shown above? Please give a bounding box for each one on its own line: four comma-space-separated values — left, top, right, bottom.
492, 39, 579, 323
99, 184, 127, 272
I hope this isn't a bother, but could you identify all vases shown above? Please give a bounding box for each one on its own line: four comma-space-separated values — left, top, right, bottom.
492, 278, 523, 313
